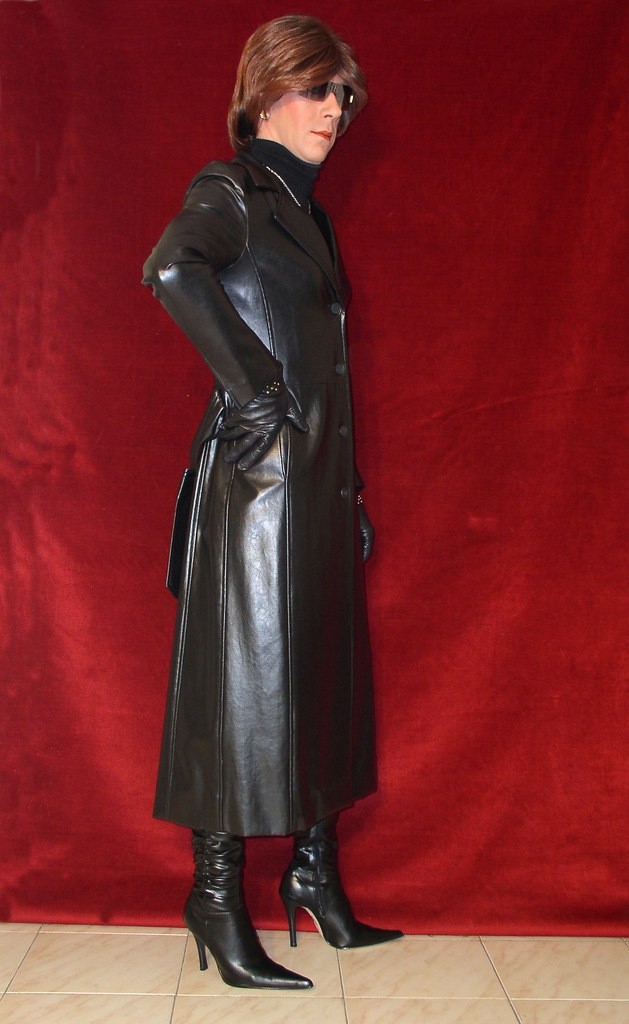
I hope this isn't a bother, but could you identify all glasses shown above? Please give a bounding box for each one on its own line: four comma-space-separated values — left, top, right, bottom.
298, 74, 360, 114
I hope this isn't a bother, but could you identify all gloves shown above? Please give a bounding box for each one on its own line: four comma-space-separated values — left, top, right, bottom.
216, 374, 312, 472
354, 493, 375, 563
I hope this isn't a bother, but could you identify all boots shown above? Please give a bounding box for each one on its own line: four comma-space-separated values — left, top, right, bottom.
183, 827, 315, 990
279, 819, 404, 951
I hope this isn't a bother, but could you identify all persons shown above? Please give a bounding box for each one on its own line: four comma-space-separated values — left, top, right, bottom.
142, 15, 404, 989
